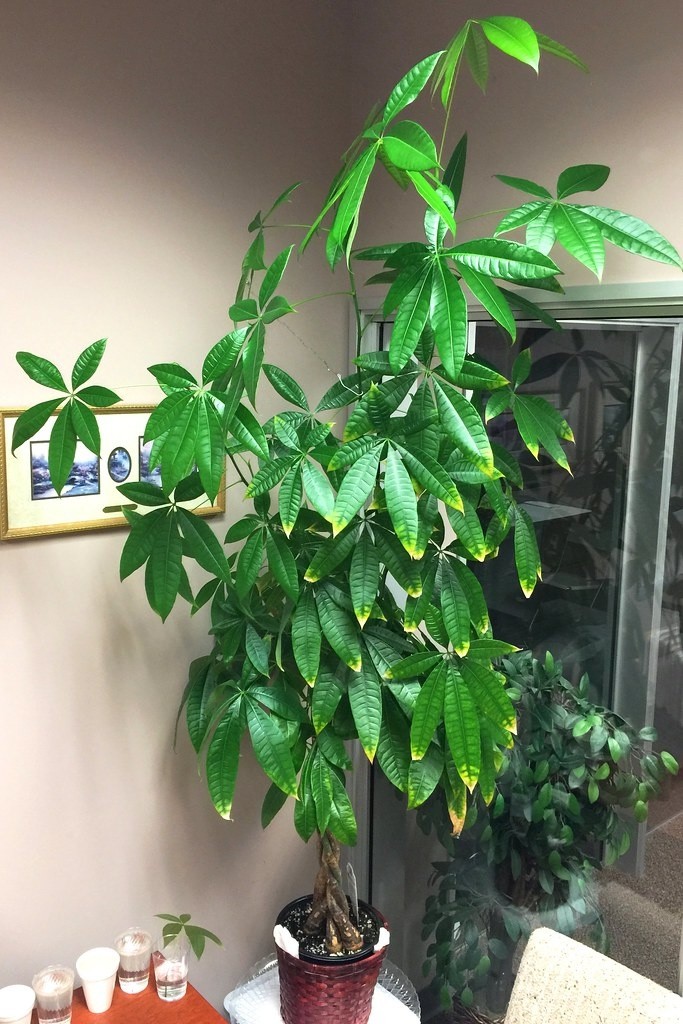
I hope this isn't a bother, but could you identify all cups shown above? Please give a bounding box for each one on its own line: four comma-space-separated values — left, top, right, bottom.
151, 934, 190, 1001
76, 947, 120, 1014
0, 984, 36, 1024
31, 965, 74, 1024
115, 926, 152, 994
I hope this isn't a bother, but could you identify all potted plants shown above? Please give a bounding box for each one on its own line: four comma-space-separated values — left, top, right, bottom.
12, 17, 682, 1023
420, 648, 680, 1013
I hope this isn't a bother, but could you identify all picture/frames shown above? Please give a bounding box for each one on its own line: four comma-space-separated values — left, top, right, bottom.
0, 400, 226, 539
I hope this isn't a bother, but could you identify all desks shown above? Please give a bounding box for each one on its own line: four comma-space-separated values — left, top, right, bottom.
30, 950, 227, 1023
